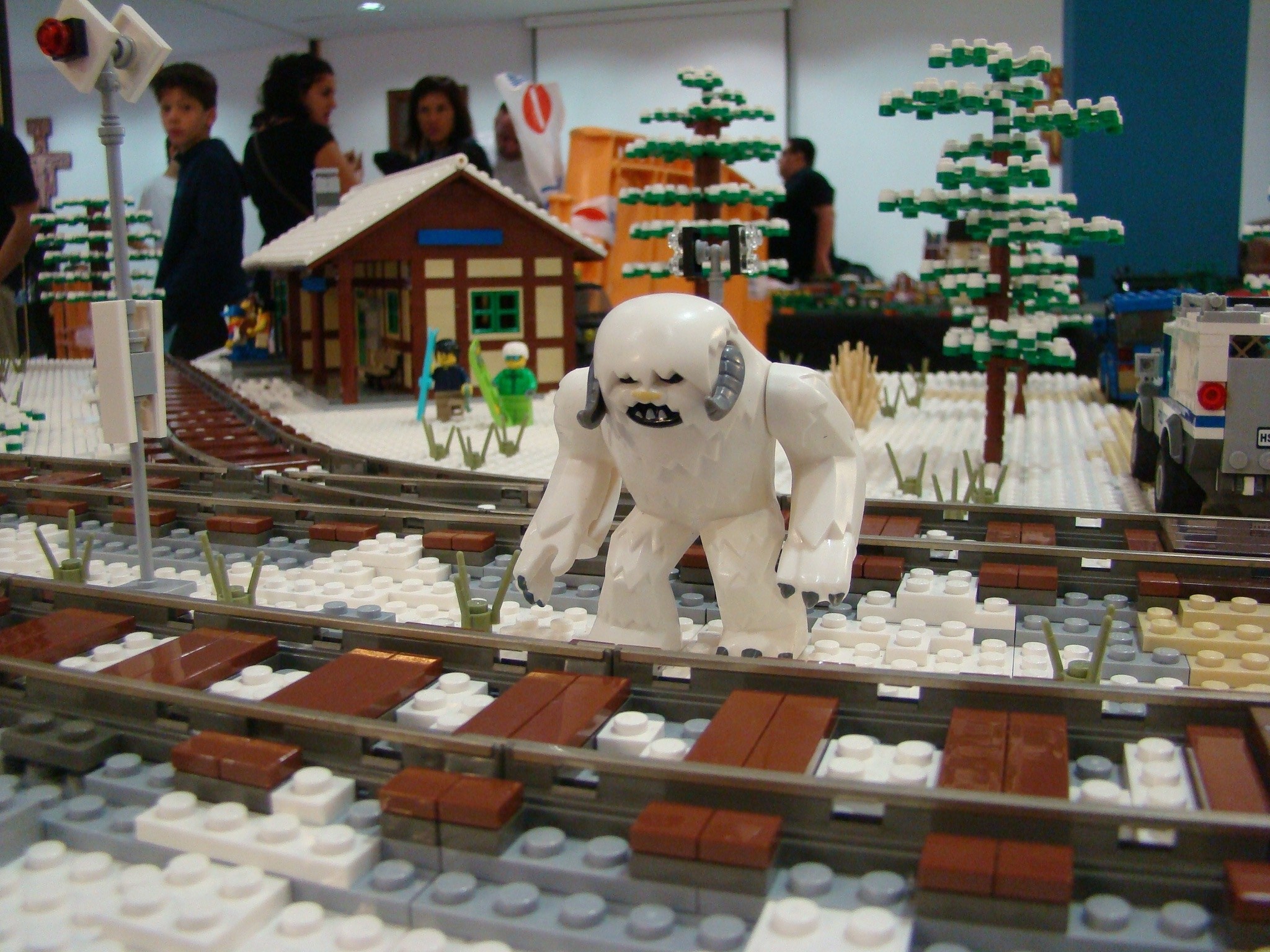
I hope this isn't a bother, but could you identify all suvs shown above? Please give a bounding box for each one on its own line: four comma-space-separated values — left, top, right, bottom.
1125, 290, 1270, 515
1089, 289, 1196, 403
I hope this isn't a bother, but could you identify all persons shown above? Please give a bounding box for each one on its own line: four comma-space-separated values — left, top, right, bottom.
0, 123, 38, 359
243, 53, 365, 311
221, 300, 271, 350
392, 75, 540, 203
418, 339, 473, 422
492, 341, 538, 427
137, 61, 249, 363
778, 137, 835, 284
